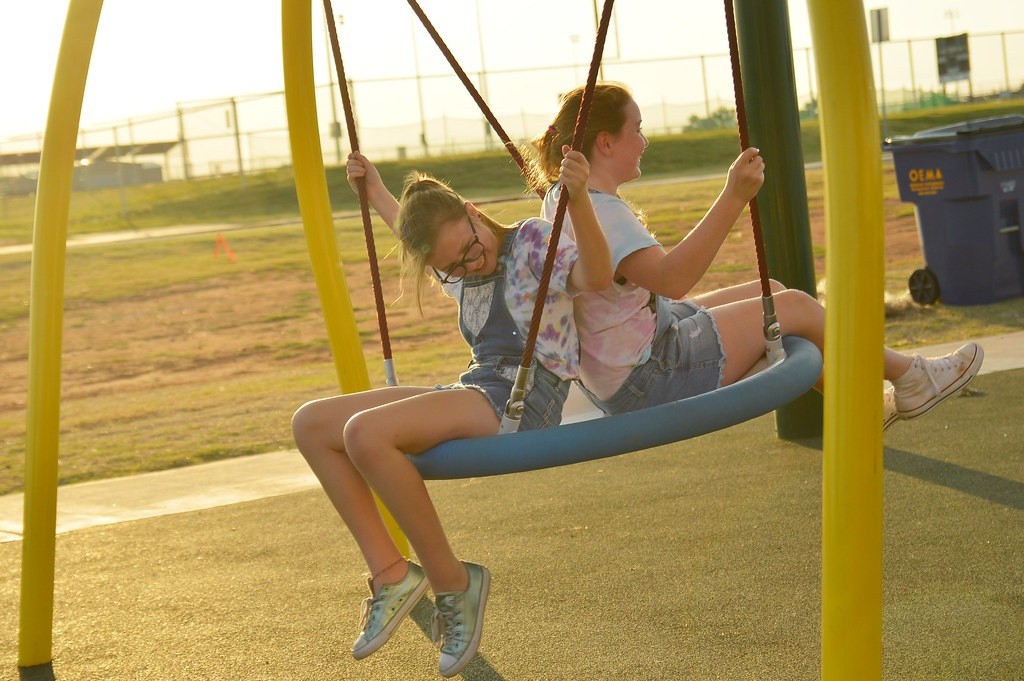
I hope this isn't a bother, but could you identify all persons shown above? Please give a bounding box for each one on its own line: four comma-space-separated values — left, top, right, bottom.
540, 83, 985, 432
288, 145, 615, 678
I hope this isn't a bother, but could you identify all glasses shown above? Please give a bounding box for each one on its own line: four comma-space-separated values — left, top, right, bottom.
432, 205, 484, 284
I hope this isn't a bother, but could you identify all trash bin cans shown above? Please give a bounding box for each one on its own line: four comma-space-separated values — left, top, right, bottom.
881, 115, 1024, 306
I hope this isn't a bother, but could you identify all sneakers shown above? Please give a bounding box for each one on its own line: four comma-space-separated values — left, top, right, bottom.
431, 560, 490, 678
883, 342, 984, 432
351, 560, 431, 660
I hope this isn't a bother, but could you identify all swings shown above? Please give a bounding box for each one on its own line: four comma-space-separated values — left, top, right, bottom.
317, 0, 824, 478
